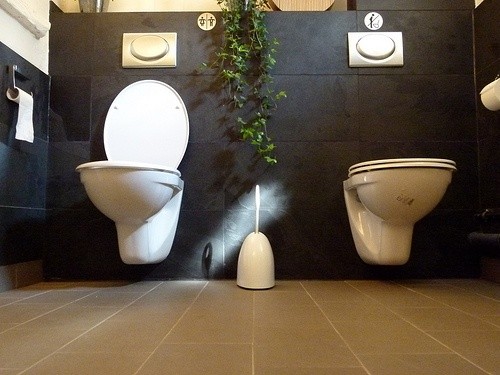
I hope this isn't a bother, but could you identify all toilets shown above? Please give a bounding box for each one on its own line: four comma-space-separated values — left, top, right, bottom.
74, 79, 191, 265
342, 157, 458, 266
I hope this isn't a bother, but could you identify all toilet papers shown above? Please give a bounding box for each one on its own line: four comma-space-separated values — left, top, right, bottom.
6, 86, 34, 143
479, 78, 500, 111
